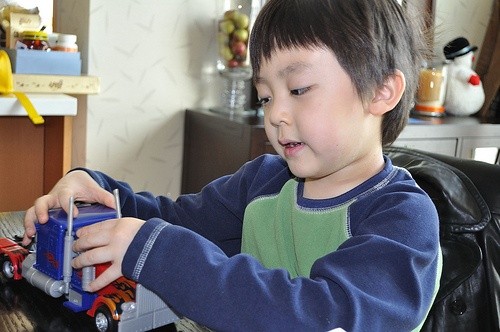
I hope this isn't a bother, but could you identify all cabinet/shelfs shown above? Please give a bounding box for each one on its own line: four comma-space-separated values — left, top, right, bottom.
180, 107, 500, 196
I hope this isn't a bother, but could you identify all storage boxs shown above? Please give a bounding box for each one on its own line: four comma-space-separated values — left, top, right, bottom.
8, 49, 81, 75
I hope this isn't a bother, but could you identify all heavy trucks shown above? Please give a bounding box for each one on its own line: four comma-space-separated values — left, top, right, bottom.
0, 188, 180, 332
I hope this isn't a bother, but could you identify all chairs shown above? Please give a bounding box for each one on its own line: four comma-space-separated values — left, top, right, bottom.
384, 146, 500, 332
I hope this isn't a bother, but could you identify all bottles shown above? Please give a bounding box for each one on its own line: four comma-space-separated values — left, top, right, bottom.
48, 32, 60, 50
56, 33, 78, 52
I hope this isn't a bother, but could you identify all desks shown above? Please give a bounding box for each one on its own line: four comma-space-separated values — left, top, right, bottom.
0, 93, 77, 212
0, 209, 214, 332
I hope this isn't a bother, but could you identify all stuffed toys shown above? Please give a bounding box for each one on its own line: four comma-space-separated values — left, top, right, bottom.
441, 38, 487, 118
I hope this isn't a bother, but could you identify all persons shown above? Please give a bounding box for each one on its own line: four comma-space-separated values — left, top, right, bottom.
22, 0, 443, 332
